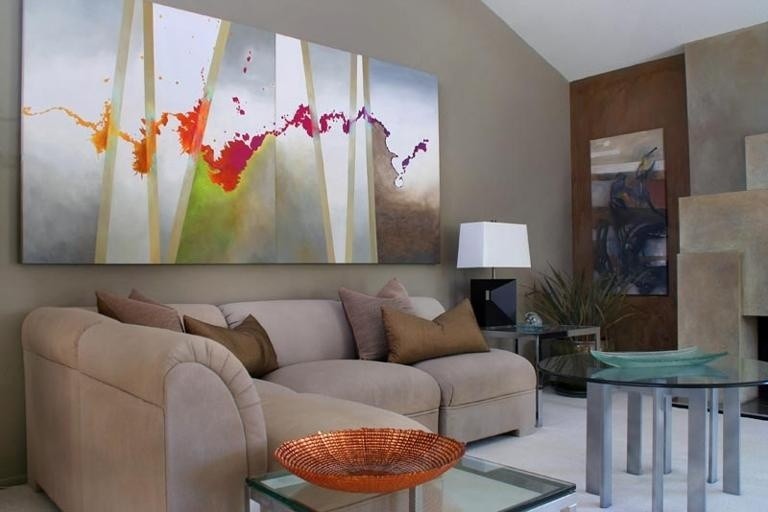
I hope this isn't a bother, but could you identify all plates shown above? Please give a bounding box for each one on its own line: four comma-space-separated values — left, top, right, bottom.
590, 365, 730, 381
588, 344, 728, 368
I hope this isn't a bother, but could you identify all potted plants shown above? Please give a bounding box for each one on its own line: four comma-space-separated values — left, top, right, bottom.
521, 255, 652, 397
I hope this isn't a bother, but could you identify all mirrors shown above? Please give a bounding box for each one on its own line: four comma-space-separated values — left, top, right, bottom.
587, 126, 671, 296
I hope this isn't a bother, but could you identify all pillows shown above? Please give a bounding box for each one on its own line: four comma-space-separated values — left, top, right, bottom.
338, 277, 417, 363
128, 287, 174, 308
380, 297, 494, 365
183, 313, 279, 378
95, 288, 184, 333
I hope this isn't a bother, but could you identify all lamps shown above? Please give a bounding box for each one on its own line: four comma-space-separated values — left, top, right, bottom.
457, 221, 531, 330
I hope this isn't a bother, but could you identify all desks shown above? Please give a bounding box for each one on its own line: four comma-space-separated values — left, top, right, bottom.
539, 358, 767, 511
482, 325, 601, 428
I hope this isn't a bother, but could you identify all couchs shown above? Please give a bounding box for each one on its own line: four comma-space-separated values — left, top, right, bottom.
20, 297, 537, 512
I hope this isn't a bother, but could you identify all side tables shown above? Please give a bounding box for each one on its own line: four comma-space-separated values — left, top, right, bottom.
245, 455, 578, 512
